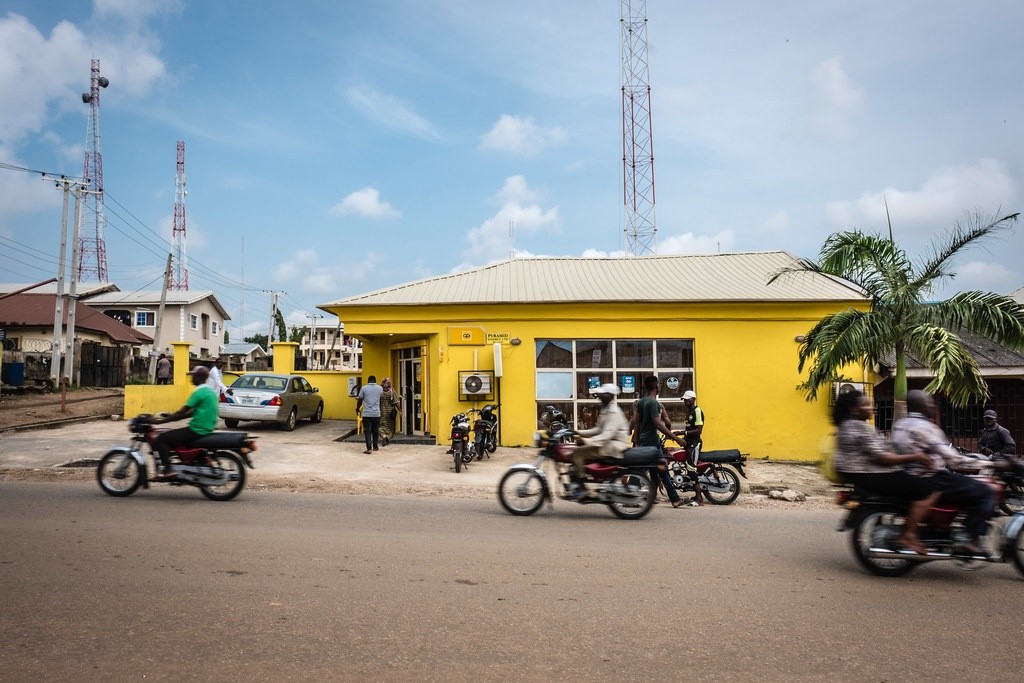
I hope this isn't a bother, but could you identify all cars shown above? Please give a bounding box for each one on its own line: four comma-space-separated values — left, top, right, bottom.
215, 374, 324, 431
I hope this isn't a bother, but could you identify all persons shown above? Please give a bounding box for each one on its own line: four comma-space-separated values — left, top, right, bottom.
561, 383, 631, 499
354, 375, 384, 454
155, 353, 172, 385
626, 386, 671, 503
626, 374, 692, 509
888, 391, 1007, 556
142, 365, 218, 479
668, 390, 705, 508
378, 378, 402, 446
829, 390, 942, 556
978, 407, 1019, 467
205, 358, 234, 397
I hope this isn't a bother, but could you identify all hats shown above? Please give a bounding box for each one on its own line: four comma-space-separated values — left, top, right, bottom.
644, 376, 657, 383
680, 390, 696, 400
186, 366, 209, 375
983, 410, 997, 418
592, 383, 621, 395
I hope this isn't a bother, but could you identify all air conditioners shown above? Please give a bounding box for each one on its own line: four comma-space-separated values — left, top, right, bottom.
461, 375, 490, 395
832, 382, 864, 405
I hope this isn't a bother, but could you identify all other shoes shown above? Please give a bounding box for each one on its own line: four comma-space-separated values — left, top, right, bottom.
156, 466, 177, 476
570, 490, 590, 496
363, 450, 371, 453
900, 534, 927, 554
373, 447, 378, 451
953, 542, 994, 556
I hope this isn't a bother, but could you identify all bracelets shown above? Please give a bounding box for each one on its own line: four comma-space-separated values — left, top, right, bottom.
685, 430, 687, 436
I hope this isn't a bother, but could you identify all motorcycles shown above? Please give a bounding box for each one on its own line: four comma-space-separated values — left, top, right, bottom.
543, 407, 569, 443
446, 412, 476, 473
95, 411, 260, 501
824, 444, 1024, 580
495, 429, 668, 520
466, 403, 502, 461
658, 430, 751, 506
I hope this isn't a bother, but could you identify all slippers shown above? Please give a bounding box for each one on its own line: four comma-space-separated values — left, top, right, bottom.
674, 497, 691, 508
688, 496, 704, 506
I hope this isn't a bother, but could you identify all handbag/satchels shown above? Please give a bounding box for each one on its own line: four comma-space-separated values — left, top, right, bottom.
823, 452, 839, 482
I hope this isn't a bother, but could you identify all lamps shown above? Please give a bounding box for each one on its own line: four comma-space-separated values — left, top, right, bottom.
796, 336, 805, 342
510, 339, 521, 345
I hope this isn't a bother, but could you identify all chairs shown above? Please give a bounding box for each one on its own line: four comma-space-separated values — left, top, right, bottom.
257, 381, 266, 386
